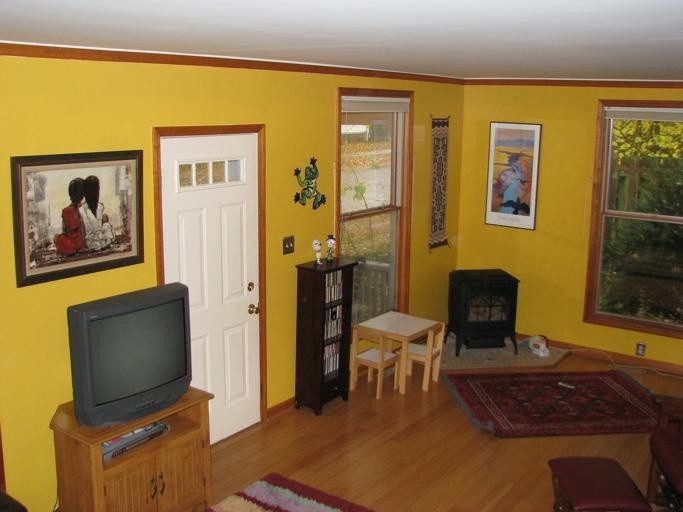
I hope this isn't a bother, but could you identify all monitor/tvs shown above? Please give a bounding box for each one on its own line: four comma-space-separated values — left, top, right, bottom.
66, 282, 192, 426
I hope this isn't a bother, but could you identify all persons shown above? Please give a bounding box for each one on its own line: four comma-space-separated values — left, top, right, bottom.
52, 177, 84, 256
79, 175, 117, 250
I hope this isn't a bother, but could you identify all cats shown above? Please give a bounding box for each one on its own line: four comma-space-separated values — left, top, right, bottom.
528, 334, 550, 358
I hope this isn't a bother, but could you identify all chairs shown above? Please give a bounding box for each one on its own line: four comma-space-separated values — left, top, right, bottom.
647, 393, 682, 488
395, 321, 446, 392
353, 327, 401, 402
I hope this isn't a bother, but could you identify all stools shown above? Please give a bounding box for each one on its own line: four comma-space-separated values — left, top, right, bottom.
549, 454, 653, 510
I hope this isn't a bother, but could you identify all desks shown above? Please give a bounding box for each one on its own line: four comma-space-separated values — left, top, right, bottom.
355, 309, 440, 395
649, 432, 683, 511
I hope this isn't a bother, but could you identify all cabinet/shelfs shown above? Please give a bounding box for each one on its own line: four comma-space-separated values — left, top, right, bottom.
295, 256, 357, 418
50, 383, 217, 512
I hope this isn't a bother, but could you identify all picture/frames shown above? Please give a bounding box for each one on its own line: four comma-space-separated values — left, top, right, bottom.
11, 148, 144, 288
483, 121, 542, 231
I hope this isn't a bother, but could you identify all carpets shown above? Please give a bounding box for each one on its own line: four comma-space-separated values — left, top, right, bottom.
417, 333, 571, 372
436, 367, 675, 437
197, 473, 377, 512
